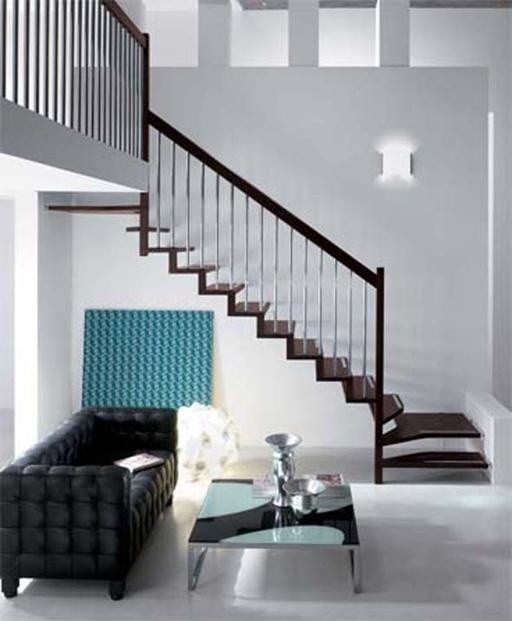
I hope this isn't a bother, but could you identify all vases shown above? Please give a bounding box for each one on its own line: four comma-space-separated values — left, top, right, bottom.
283, 479, 325, 516
266, 434, 302, 509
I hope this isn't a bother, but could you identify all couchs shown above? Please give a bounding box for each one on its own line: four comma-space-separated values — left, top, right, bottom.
0, 408, 178, 597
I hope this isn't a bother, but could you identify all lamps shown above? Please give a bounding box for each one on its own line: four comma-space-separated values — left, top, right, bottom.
382, 152, 411, 175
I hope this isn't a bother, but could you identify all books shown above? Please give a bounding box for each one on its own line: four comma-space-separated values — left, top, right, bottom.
309, 475, 348, 504
112, 453, 165, 473
